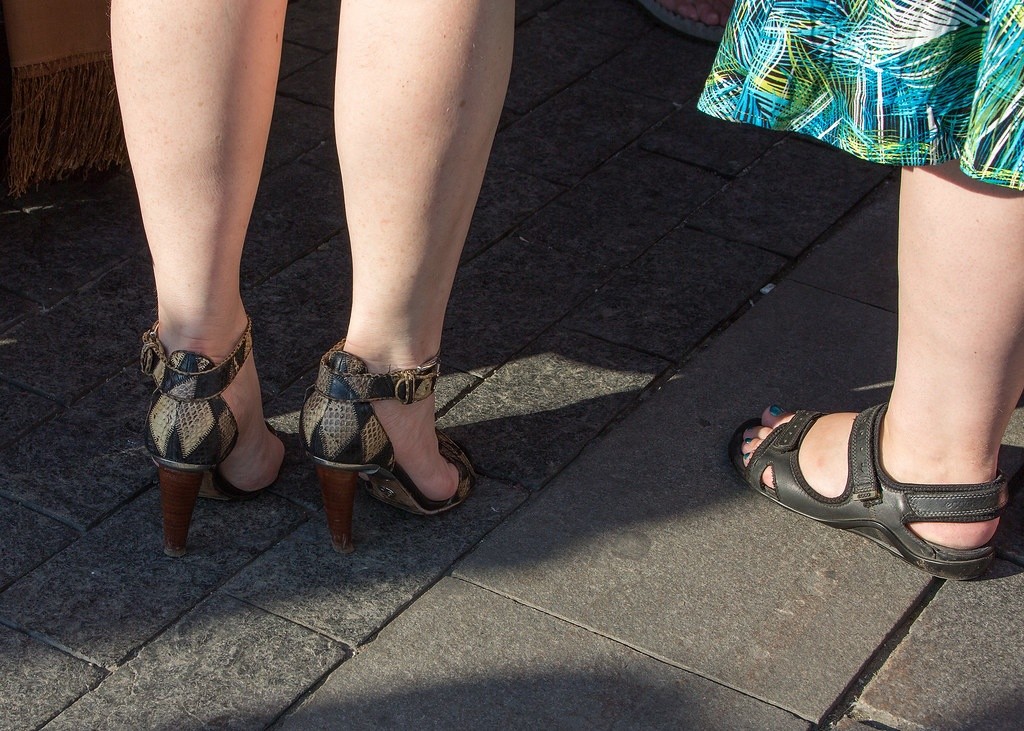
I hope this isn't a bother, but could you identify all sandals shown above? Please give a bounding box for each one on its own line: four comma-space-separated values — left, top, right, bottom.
728, 404, 1006, 581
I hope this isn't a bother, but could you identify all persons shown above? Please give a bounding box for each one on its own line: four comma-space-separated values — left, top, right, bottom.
106, 0, 485, 557
643, 0, 743, 37
696, 0, 1023, 583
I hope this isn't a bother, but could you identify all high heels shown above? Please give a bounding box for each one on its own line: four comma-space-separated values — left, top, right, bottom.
298, 338, 480, 554
138, 315, 290, 558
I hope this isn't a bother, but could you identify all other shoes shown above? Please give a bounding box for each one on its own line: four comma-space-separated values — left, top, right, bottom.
643, 0, 725, 42
